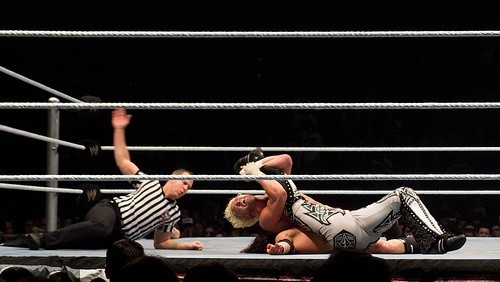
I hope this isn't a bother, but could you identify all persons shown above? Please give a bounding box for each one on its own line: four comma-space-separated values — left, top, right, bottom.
24, 108, 201, 250
0, 212, 223, 246
404, 216, 499, 237
224, 146, 467, 255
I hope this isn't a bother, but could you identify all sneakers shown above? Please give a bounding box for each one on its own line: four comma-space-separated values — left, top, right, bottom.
429, 233, 466, 256
24, 232, 42, 250
234, 150, 263, 170
